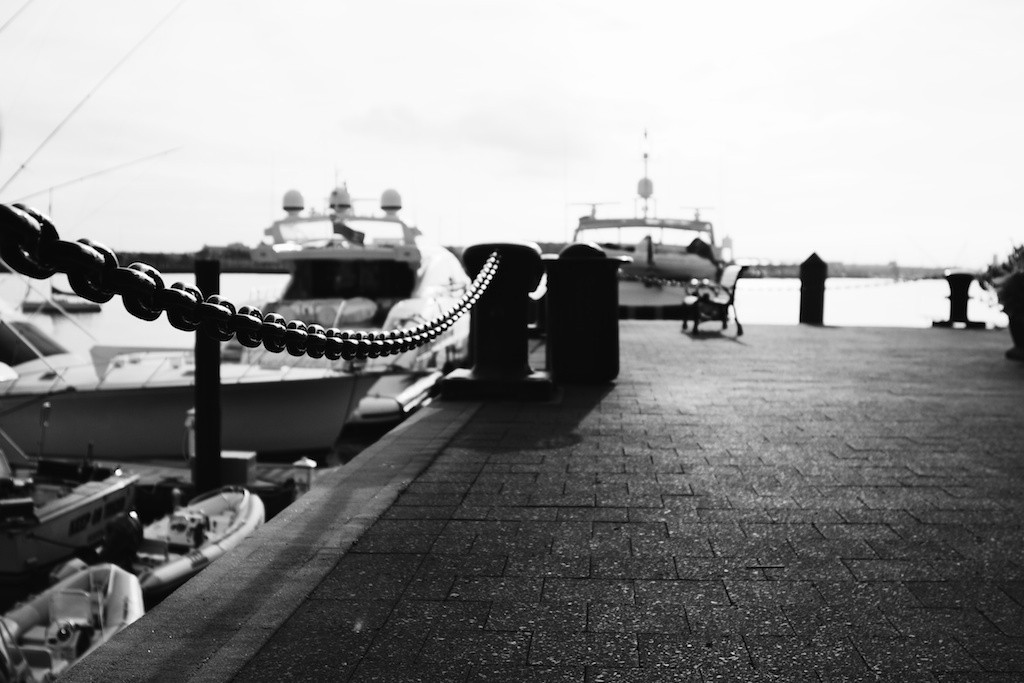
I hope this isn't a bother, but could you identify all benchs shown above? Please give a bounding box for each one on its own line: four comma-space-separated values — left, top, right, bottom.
678, 261, 751, 339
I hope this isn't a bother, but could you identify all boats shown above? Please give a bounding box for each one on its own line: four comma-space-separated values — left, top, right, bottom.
572, 124, 722, 319
0, 180, 470, 682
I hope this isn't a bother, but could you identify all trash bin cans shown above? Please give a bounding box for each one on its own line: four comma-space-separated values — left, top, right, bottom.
544, 243, 633, 378
